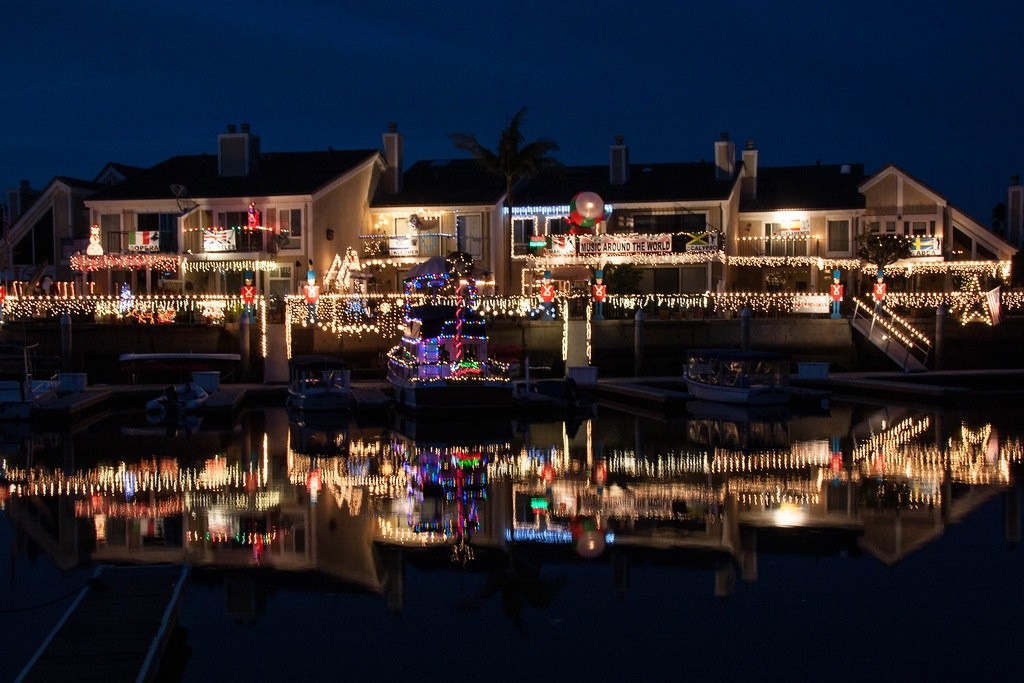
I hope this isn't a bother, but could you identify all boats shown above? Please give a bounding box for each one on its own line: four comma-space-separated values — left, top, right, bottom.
511, 357, 580, 404
117, 352, 250, 439
283, 354, 364, 427
681, 347, 778, 406
0, 340, 116, 438
386, 255, 519, 425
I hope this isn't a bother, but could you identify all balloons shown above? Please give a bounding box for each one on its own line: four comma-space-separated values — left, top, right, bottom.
561, 193, 605, 227
576, 192, 603, 218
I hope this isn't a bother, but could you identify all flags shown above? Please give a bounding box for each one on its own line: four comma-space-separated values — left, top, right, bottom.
128, 231, 160, 251
204, 229, 236, 251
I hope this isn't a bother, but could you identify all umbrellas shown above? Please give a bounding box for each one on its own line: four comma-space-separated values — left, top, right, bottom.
406, 256, 493, 278
